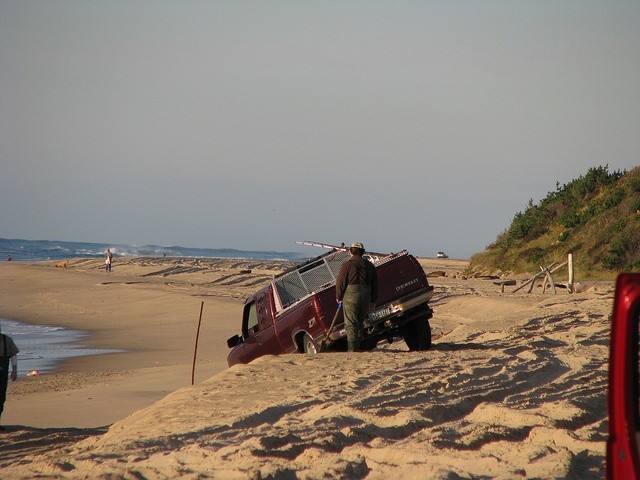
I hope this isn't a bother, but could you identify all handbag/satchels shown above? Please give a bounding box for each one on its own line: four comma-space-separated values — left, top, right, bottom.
105, 256, 110, 265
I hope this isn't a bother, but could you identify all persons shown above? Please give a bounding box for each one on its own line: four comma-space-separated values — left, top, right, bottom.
0, 329, 18, 423
6, 254, 11, 260
105, 248, 113, 272
336, 242, 378, 353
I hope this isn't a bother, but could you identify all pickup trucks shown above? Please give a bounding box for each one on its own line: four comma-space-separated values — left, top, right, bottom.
227, 242, 434, 370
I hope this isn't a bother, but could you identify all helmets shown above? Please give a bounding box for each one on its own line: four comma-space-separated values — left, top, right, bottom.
351, 241, 365, 250
